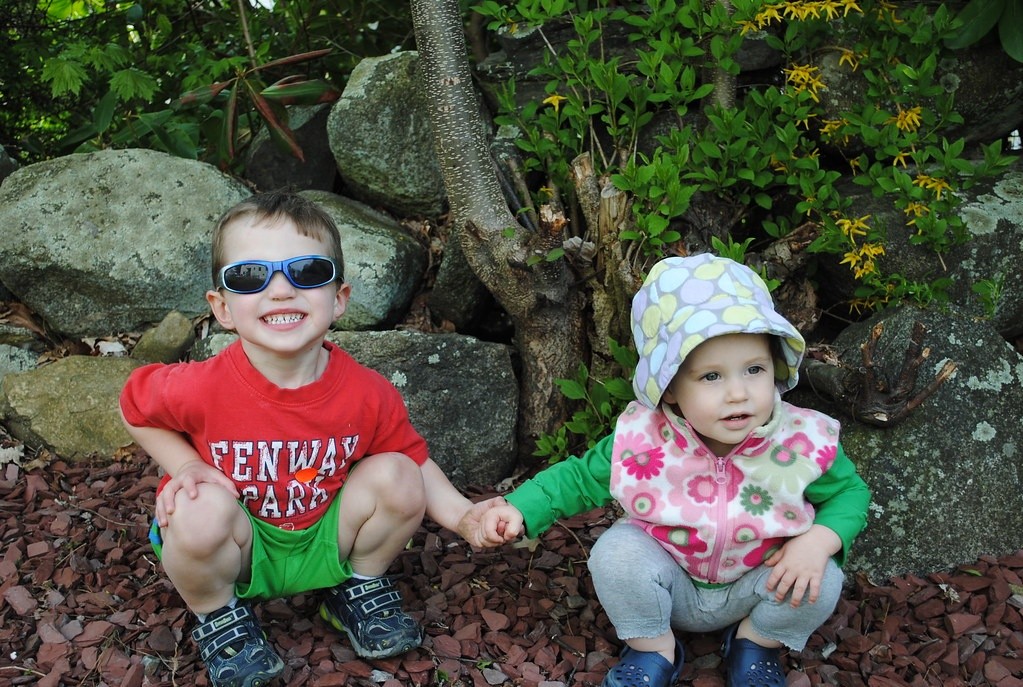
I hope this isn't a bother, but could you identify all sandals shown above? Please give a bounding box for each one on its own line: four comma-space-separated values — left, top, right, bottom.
601, 638, 685, 687
720, 621, 788, 687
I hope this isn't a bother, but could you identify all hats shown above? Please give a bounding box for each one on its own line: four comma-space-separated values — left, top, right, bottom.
631, 253, 806, 411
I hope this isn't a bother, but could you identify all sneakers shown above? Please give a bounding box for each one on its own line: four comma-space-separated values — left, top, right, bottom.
320, 578, 423, 661
191, 599, 284, 687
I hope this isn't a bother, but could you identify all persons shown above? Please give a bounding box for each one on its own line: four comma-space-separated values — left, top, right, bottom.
117, 189, 526, 687
475, 253, 873, 687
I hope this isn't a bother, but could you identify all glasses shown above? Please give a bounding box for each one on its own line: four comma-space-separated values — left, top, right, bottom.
216, 255, 345, 294
